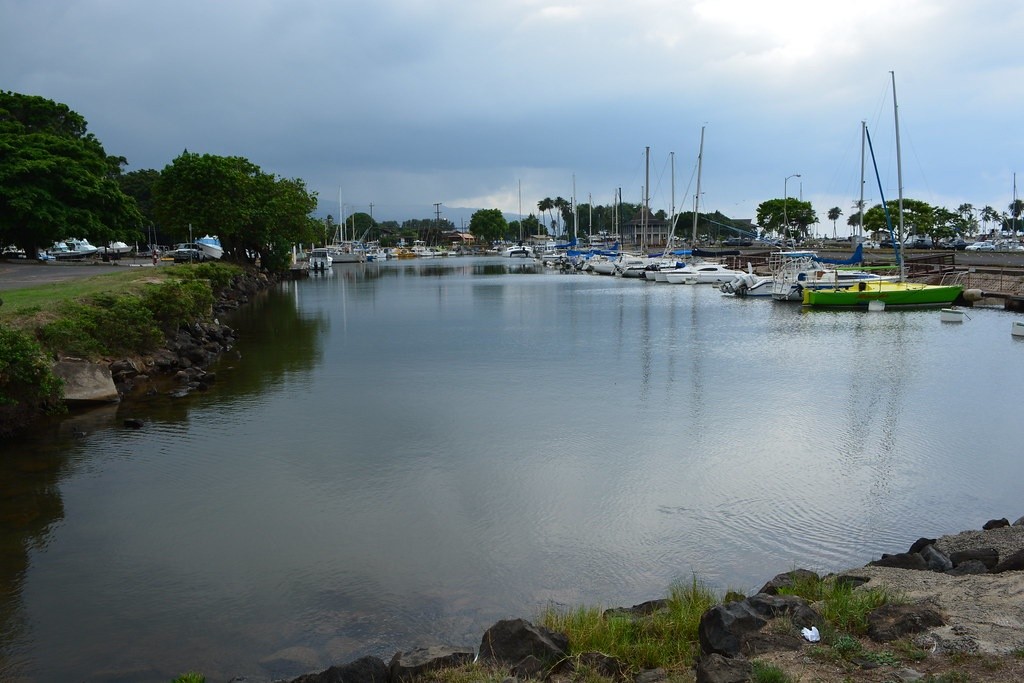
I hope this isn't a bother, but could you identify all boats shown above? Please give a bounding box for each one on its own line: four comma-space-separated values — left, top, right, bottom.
1, 236, 133, 262
196, 233, 226, 259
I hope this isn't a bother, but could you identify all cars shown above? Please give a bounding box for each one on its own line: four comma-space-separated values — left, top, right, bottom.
965, 242, 997, 252
952, 239, 968, 253
862, 240, 880, 250
723, 238, 753, 247
173, 243, 207, 264
938, 241, 955, 251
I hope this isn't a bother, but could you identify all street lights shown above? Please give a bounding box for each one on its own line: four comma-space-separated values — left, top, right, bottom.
782, 174, 801, 246
692, 192, 705, 229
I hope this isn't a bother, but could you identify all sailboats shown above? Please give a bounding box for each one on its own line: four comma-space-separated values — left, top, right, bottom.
288, 69, 966, 307
